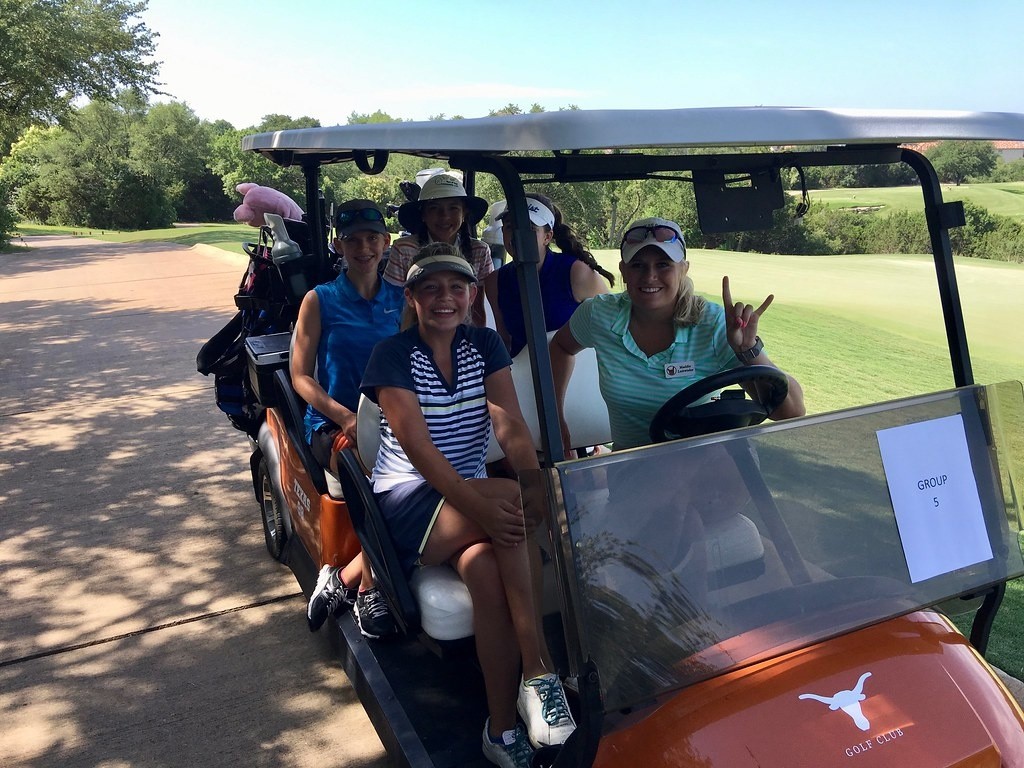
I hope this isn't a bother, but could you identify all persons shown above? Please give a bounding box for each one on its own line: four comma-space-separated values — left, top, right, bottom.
290, 173, 807, 768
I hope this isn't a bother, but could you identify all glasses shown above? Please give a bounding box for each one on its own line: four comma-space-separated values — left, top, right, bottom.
620, 225, 687, 262
335, 208, 388, 229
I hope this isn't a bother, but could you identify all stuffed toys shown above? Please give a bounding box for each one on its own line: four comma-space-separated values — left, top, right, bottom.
233, 182, 304, 227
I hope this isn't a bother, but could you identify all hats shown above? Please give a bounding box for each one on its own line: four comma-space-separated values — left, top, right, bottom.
622, 217, 686, 263
402, 255, 478, 288
398, 175, 488, 234
335, 199, 387, 241
495, 197, 555, 229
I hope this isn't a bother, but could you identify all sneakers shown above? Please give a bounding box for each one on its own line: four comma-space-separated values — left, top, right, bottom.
306, 564, 347, 634
353, 585, 398, 640
482, 715, 534, 768
516, 672, 578, 749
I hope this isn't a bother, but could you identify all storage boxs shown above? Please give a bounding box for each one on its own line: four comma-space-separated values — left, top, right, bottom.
244, 329, 292, 405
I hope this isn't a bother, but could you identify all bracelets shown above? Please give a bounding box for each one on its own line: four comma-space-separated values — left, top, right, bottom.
735, 335, 765, 363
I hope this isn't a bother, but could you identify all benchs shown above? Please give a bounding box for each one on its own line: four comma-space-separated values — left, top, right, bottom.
272, 288, 498, 501
335, 330, 768, 644
